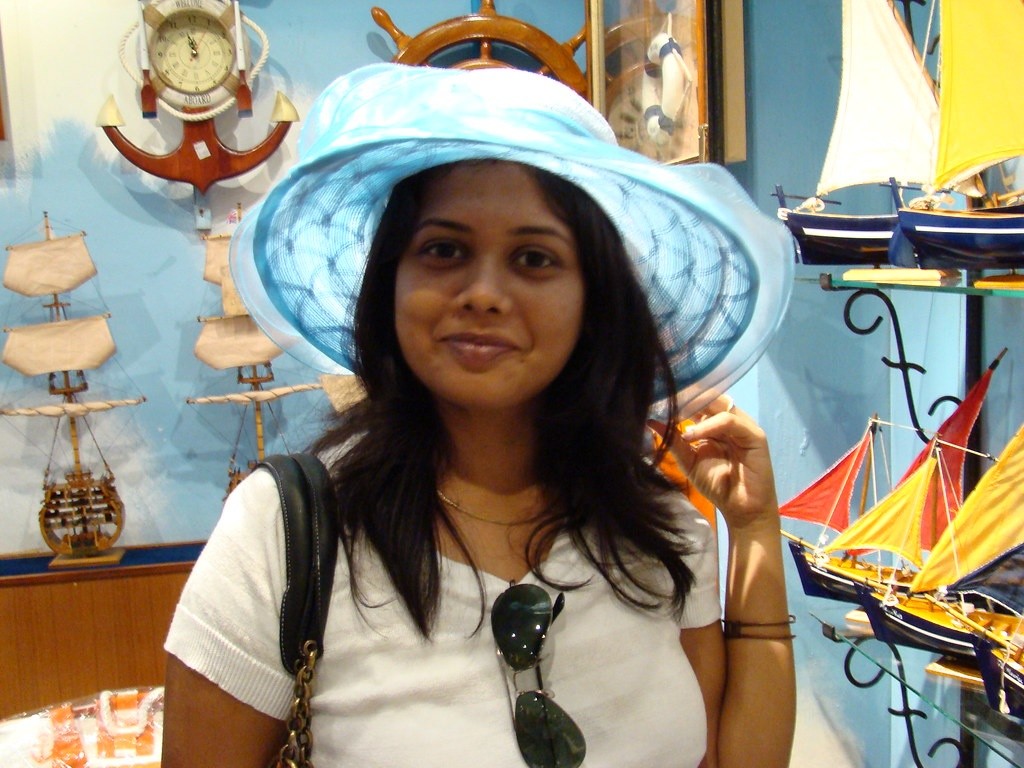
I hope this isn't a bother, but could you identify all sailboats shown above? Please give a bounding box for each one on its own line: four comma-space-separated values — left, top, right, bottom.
0, 207, 146, 571
770, 0, 1021, 275
196, 202, 366, 510
773, 346, 1024, 723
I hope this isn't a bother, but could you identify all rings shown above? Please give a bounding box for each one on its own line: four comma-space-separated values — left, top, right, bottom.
728, 404, 736, 414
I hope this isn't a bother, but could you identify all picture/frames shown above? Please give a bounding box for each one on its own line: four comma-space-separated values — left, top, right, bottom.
583, 0, 709, 166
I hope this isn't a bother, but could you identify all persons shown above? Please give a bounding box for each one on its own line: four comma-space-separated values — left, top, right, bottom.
161, 62, 796, 768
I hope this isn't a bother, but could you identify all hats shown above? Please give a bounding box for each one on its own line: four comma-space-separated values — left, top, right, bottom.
228, 62, 794, 428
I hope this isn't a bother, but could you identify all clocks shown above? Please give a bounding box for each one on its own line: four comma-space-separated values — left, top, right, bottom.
149, 9, 236, 94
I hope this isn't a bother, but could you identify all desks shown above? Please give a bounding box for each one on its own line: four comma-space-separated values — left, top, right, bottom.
0, 539, 211, 726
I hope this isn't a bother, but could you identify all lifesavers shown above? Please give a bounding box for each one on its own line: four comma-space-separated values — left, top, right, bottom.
882, 594, 898, 606
818, 553, 830, 563
801, 198, 825, 212
640, 33, 686, 141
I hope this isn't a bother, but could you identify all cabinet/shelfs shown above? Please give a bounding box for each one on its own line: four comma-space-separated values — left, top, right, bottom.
793, 275, 1024, 767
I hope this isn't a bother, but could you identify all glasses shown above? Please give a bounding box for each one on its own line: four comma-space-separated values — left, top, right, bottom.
490, 578, 586, 768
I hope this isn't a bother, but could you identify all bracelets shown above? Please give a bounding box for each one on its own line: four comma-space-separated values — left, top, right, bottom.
722, 615, 797, 640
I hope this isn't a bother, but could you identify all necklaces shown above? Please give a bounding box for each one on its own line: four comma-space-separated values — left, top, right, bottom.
437, 490, 555, 525
